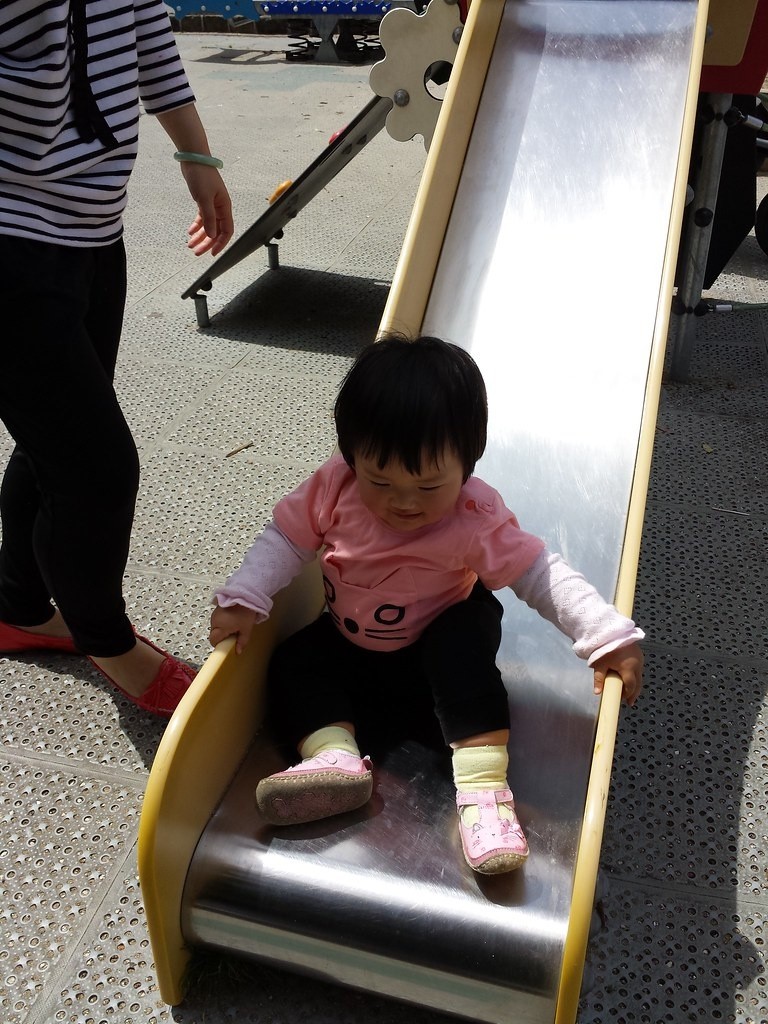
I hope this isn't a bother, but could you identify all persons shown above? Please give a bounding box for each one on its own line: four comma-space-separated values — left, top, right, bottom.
0, 0, 238, 729
204, 322, 645, 877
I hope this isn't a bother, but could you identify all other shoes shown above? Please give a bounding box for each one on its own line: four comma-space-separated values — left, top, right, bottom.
0, 622, 81, 654
452, 787, 530, 875
88, 628, 201, 719
257, 749, 376, 825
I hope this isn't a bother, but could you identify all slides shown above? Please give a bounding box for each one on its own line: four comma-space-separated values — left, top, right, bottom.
126, 1, 724, 1024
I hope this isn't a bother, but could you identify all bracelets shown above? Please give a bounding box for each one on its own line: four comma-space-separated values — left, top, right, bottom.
173, 150, 224, 170
